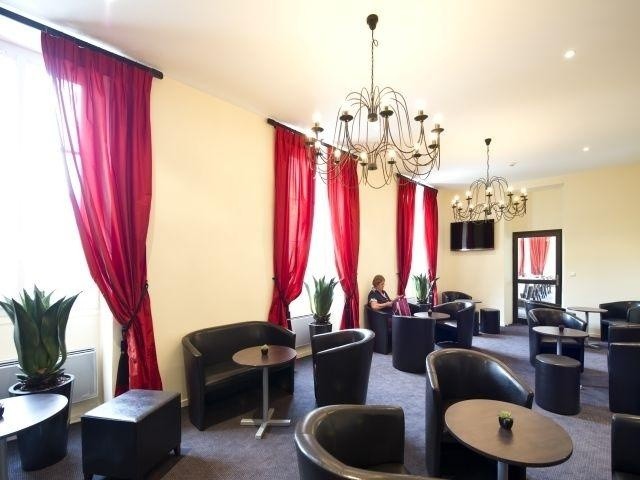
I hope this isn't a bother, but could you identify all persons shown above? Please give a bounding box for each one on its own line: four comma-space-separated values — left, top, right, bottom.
368, 275, 400, 333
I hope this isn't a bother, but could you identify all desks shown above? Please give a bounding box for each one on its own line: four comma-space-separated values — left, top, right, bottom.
0, 390, 70, 479
444, 399, 574, 479
229, 341, 298, 440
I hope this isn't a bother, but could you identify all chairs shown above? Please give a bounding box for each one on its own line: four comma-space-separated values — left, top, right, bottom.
179, 318, 297, 432
517, 278, 556, 302
290, 402, 441, 480
310, 326, 378, 409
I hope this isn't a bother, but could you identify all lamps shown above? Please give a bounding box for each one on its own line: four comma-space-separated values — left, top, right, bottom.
304, 12, 445, 194
448, 136, 530, 226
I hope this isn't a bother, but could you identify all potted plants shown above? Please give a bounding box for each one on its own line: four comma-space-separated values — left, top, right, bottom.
410, 272, 442, 312
0, 282, 89, 474
299, 273, 345, 369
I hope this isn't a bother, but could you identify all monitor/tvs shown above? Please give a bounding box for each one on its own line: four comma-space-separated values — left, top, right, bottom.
449, 218, 495, 251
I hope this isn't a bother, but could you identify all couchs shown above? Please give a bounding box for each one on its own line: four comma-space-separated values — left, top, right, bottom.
526, 300, 640, 479
364, 291, 502, 374
425, 349, 535, 478
294, 404, 427, 478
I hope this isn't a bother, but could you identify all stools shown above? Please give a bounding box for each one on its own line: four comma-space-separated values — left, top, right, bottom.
78, 389, 183, 480
